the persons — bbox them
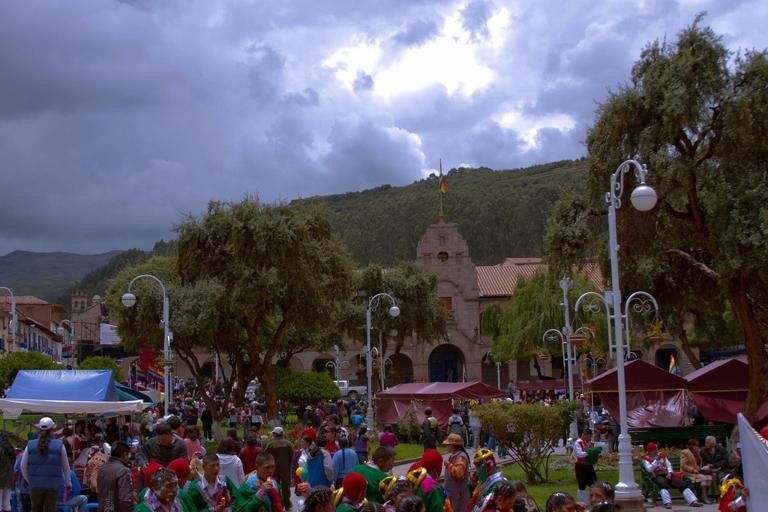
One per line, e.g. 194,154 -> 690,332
1,345 -> 767,512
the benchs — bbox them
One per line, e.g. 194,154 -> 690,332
639,457 -> 702,504
626,424 -> 732,449
229,414 -> 263,429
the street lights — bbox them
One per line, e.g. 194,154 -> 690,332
371,327 -> 396,393
580,349 -> 606,377
358,293 -> 399,433
56,320 -> 75,371
484,352 -> 501,390
122,274 -> 172,415
324,344 -> 350,386
0,285 -> 17,351
573,156 -> 660,511
541,274 -> 596,410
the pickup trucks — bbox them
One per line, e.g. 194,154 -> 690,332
332,380 -> 367,400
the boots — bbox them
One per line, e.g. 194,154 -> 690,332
701,486 -> 712,504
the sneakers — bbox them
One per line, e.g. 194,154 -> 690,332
665,503 -> 671,509
690,501 -> 704,507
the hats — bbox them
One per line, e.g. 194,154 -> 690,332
271,427 -> 283,435
40,417 -> 55,431
331,433 -> 494,503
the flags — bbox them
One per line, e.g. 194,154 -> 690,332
439,159 -> 448,193
